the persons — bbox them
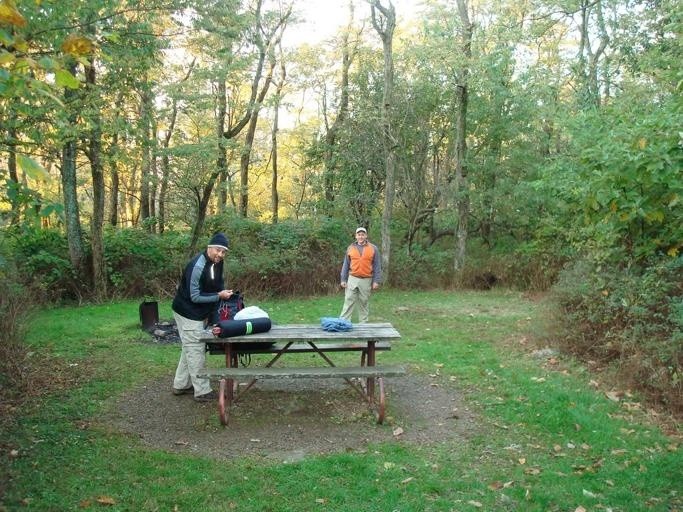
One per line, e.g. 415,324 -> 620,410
340,227 -> 381,322
171,232 -> 234,401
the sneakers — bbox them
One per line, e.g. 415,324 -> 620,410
194,390 -> 219,402
172,385 -> 194,396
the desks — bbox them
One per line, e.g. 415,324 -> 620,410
199,321 -> 403,401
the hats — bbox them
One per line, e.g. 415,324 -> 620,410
208,233 -> 229,251
356,227 -> 368,234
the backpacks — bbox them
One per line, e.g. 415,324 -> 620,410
209,288 -> 246,326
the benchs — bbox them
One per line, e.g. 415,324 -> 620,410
196,366 -> 407,426
208,340 -> 392,389
